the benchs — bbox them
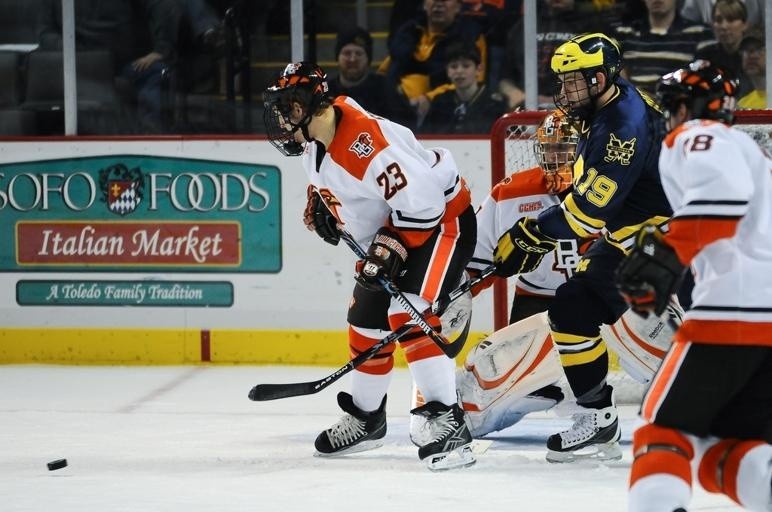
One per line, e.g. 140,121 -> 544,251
0,0 -> 772,134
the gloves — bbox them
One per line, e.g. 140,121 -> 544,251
493,216 -> 559,280
353,225 -> 414,295
614,226 -> 693,319
302,186 -> 343,246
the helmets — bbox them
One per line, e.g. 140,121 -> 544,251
649,58 -> 742,140
532,107 -> 584,194
260,57 -> 334,158
550,31 -> 625,111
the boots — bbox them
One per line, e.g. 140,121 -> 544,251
547,386 -> 623,453
314,390 -> 390,454
411,389 -> 474,462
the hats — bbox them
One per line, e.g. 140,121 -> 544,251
333,23 -> 372,62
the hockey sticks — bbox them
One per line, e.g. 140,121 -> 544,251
248,260 -> 501,402
335,227 -> 472,360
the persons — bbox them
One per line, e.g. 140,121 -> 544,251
451,107 -> 656,443
494,29 -> 695,452
611,59 -> 772,512
266,60 -> 472,458
